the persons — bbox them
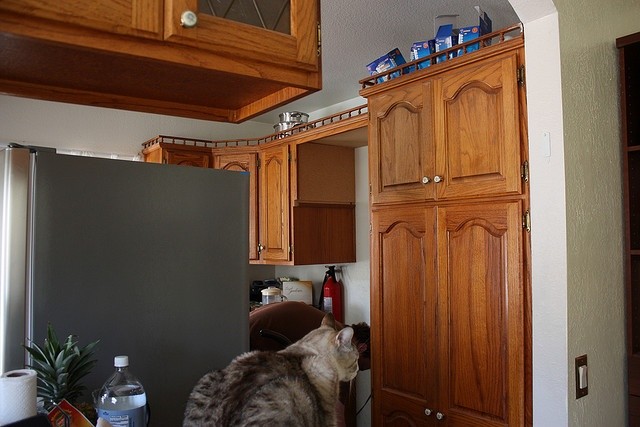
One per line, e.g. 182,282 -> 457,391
249,301 -> 371,427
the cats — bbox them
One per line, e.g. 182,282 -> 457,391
181,310 -> 360,427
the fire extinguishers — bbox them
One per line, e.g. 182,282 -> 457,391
318,265 -> 342,323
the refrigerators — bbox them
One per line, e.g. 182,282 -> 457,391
0,149 -> 251,427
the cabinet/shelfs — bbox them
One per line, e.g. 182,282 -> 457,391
0,0 -> 323,124
358,21 -> 531,205
210,147 -> 259,265
371,198 -> 532,427
146,144 -> 211,170
259,146 -> 357,267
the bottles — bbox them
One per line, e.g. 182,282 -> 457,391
98,355 -> 146,427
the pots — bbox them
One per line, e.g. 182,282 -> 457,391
278,110 -> 309,123
272,122 -> 316,139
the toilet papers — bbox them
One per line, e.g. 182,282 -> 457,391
0,368 -> 36,426
